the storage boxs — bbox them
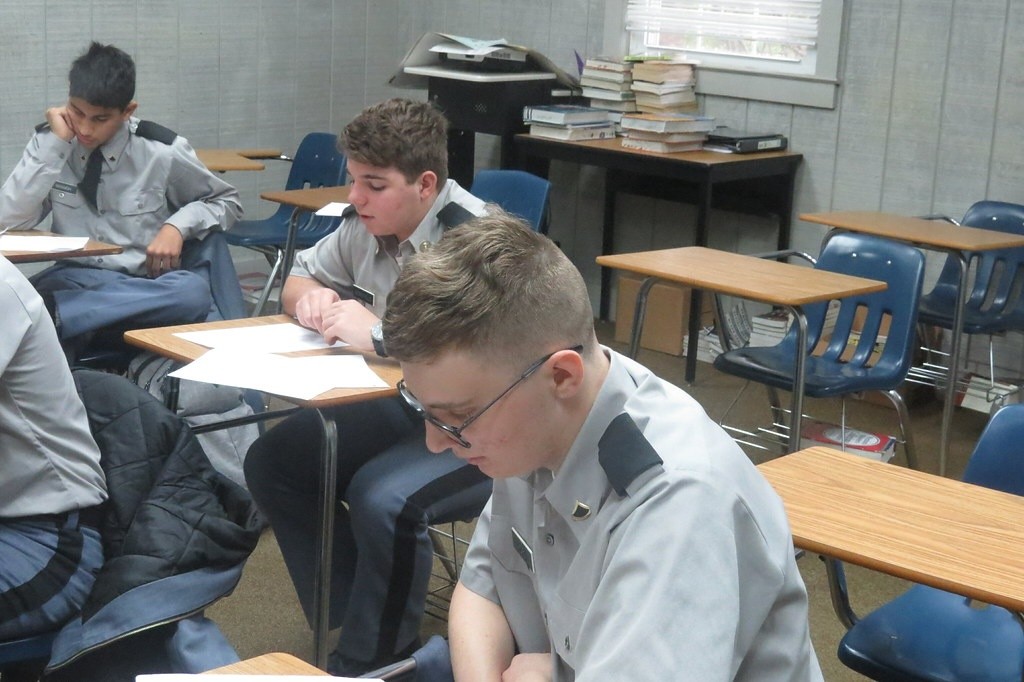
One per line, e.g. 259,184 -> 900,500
615,271 -> 714,356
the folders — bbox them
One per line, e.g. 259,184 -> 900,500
703,126 -> 788,154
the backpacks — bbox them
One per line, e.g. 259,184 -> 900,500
120,350 -> 270,533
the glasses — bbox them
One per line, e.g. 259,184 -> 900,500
396,344 -> 583,449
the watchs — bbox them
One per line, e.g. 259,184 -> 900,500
371,319 -> 390,358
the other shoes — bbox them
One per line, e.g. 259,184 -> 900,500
326,634 -> 421,681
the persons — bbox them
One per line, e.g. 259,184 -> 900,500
382,209 -> 826,682
0,38 -> 242,350
242,96 -> 507,676
0,249 -> 109,646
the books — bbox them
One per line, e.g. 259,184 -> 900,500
680,315 -> 886,365
523,52 -> 790,154
799,415 -> 898,463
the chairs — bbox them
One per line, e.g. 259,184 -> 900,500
76,231 -> 245,364
471,169 -> 551,234
224,131 -> 347,316
709,232 -> 919,472
916,200 -> 1024,401
837,404 -> 1024,682
0,370 -> 260,682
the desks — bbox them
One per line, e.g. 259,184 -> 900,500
507,132 -> 803,384
0,138 -> 1023,682
404,65 -> 584,191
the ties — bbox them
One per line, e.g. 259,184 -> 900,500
77,148 -> 106,211
